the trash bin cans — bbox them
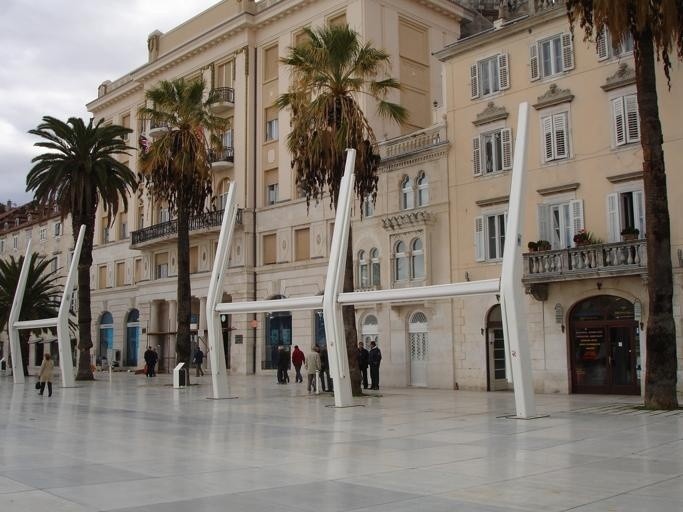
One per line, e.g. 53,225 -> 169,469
101,356 -> 107,372
173,362 -> 187,389
0,357 -> 7,376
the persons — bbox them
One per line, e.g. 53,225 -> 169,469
315,343 -> 332,391
356,341 -> 369,389
36,352 -> 54,397
148,348 -> 158,377
192,346 -> 203,377
303,345 -> 320,393
291,345 -> 305,383
366,340 -> 381,390
142,345 -> 156,377
274,345 -> 290,384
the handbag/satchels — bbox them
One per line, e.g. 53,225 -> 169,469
35,382 -> 41,390
